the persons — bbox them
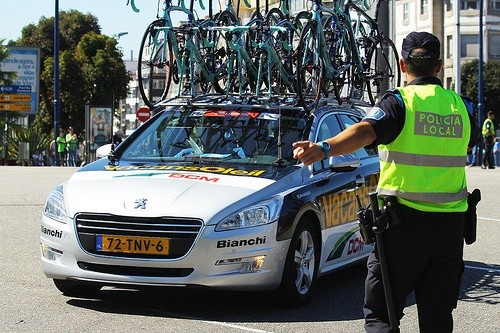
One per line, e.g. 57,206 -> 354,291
481,111 -> 497,169
492,138 -> 500,167
56,131 -> 66,167
50,139 -> 56,166
292,31 -> 481,333
65,127 -> 79,167
465,142 -> 482,167
107,129 -> 125,144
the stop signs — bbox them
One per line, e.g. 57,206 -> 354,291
136,107 -> 151,122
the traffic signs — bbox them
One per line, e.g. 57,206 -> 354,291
0,84 -> 32,112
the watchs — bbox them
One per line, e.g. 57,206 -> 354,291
318,141 -> 330,159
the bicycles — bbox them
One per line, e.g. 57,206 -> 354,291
126,0 -> 400,113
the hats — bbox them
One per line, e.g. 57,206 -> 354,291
401,31 -> 440,60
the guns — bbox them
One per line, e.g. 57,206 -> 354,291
465,189 -> 481,212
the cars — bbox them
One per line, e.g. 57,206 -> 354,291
37,88 -> 385,304
